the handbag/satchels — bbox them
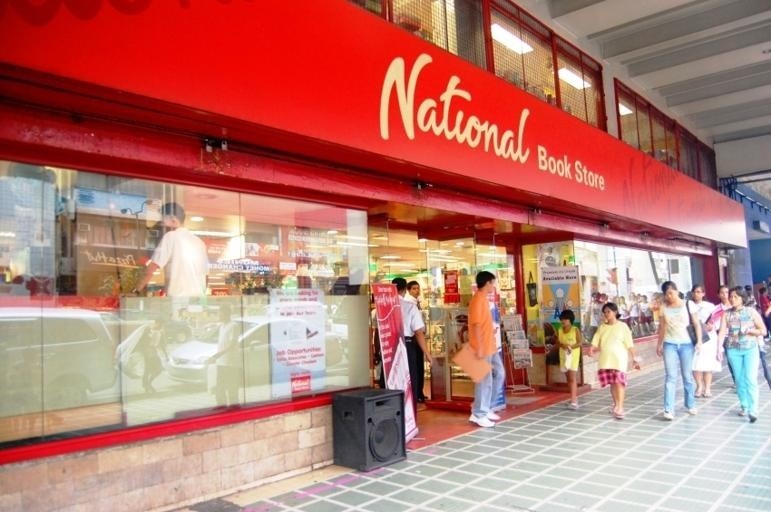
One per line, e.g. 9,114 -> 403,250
526,271 -> 538,307
686,299 -> 709,346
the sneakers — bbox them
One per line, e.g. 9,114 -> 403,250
487,411 -> 500,420
663,412 -> 673,419
468,414 -> 494,427
687,408 -> 697,415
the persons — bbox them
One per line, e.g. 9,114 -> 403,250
655,280 -> 703,422
115,199 -> 211,297
716,285 -> 768,423
584,290 -> 656,344
138,317 -> 170,397
206,302 -> 242,414
406,280 -> 430,405
467,271 -> 506,431
688,277 -> 771,398
556,309 -> 583,410
368,277 -> 433,389
586,301 -> 642,419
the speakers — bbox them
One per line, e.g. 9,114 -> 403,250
332,388 -> 407,473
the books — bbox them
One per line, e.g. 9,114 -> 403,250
452,344 -> 496,387
502,314 -> 533,370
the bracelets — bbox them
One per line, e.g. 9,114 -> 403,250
130,287 -> 142,296
